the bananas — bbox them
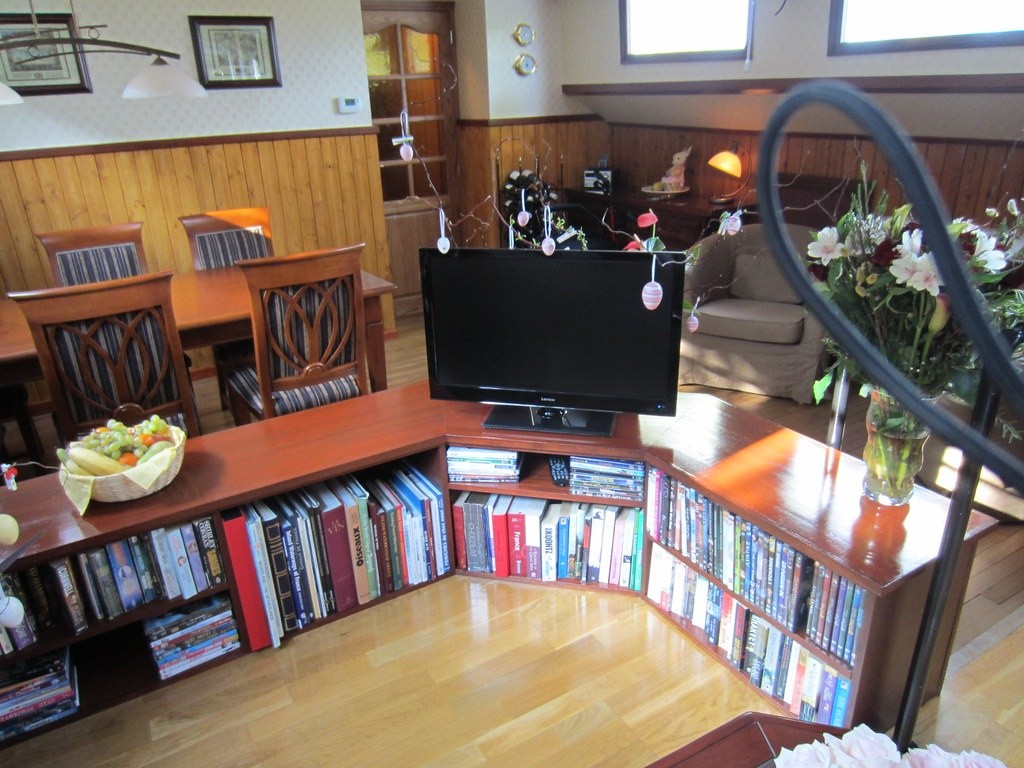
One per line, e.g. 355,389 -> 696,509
64,446 -> 134,476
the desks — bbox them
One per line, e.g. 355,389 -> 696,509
565,186 -> 756,246
0,267 -> 396,391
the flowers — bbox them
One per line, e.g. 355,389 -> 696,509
797,162 -> 1024,497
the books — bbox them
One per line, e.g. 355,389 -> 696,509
0,517 -> 226,659
646,542 -> 854,727
447,446 -> 524,483
569,456 -> 645,501
646,466 -> 866,668
453,492 -> 644,590
0,646 -> 79,742
145,592 -> 240,680
222,464 -> 451,651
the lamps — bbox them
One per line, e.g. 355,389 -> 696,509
0,24 -> 209,99
0,82 -> 25,105
708,140 -> 751,204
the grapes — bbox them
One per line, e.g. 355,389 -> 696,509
79,414 -> 171,460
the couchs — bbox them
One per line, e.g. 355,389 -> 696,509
680,222 -> 832,404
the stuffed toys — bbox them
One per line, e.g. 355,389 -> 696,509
663,146 -> 692,191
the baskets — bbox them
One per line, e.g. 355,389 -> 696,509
89,424 -> 186,503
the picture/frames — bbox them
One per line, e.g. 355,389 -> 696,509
189,15 -> 282,89
0,13 -> 93,96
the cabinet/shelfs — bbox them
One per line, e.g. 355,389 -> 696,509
641,460 -> 874,727
0,440 -> 449,749
447,443 -> 648,595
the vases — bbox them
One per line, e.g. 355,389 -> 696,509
863,383 -> 931,508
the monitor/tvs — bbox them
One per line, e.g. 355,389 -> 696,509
418,247 -> 686,437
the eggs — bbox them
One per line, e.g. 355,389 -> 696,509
0,514 -> 19,545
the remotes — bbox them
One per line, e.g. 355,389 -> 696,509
549,455 -> 570,487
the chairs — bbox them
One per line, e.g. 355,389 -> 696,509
0,381 -> 46,470
178,206 -> 274,410
223,240 -> 369,427
7,268 -> 199,440
34,223 -> 151,287
